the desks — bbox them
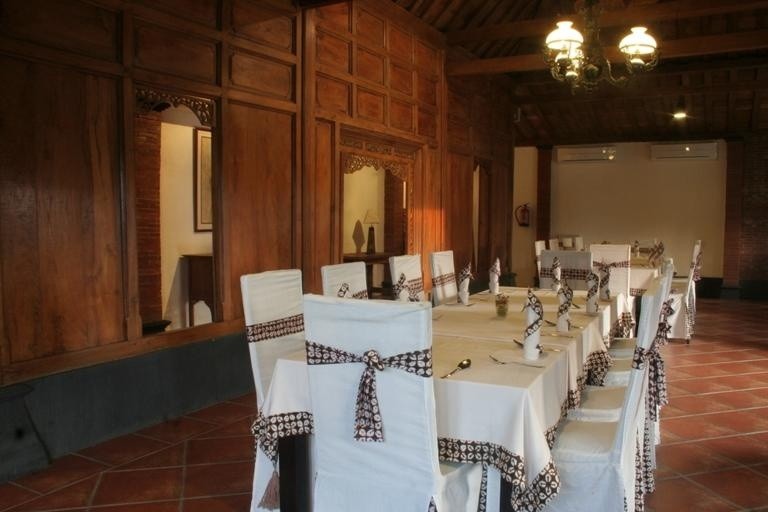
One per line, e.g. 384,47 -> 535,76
183,253 -> 215,327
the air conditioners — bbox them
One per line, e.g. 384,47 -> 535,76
648,142 -> 718,161
555,145 -> 616,164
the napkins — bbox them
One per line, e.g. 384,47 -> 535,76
338,283 -> 353,300
488,256 -> 502,296
395,272 -> 411,301
556,275 -> 574,334
522,287 -> 544,362
457,262 -> 475,306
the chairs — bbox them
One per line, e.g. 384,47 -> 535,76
305,292 -> 501,512
540,348 -> 656,512
320,261 -> 369,299
534,236 -> 701,345
430,250 -> 457,307
568,256 -> 676,493
238,268 -> 307,415
389,254 -> 426,301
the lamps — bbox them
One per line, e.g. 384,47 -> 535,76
539,0 -> 663,97
363,208 -> 381,253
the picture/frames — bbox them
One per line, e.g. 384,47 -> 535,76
192,125 -> 215,231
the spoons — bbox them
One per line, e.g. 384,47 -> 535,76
440,358 -> 471,379
